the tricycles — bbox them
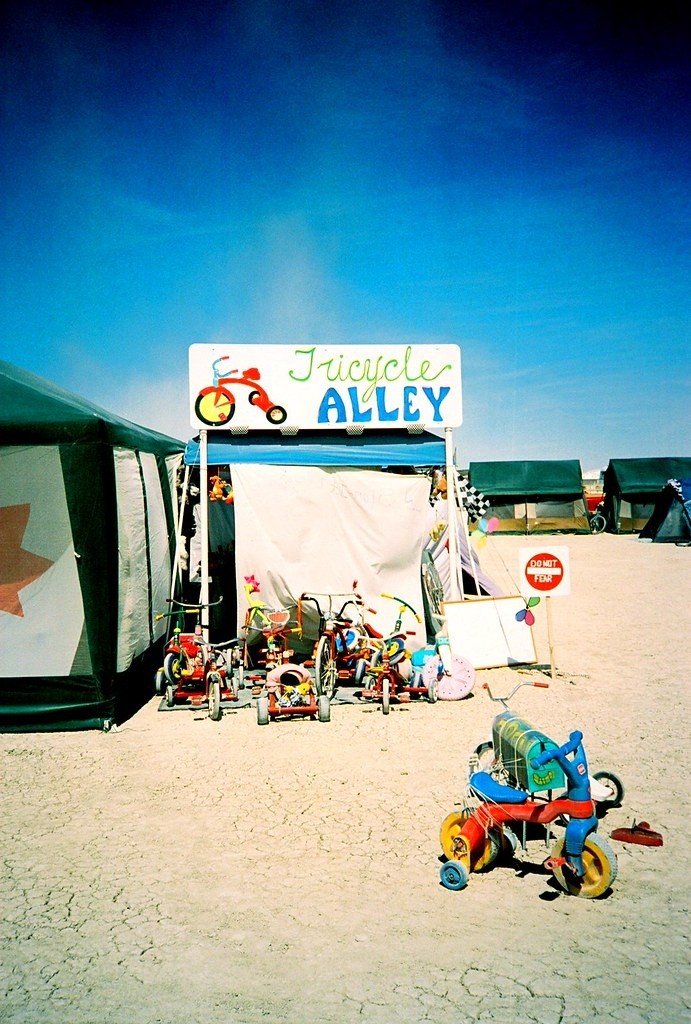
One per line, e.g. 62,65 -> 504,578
438,679 -> 625,898
153,581 -> 438,725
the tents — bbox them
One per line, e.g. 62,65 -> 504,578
602,455 -> 691,535
468,458 -> 592,536
182,427 -> 457,682
637,478 -> 691,543
0,360 -> 187,734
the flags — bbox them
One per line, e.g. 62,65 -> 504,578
455,471 -> 490,525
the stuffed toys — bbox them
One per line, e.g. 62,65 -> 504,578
207,474 -> 234,504
433,473 -> 447,499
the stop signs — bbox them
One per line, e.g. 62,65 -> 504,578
519,544 -> 572,598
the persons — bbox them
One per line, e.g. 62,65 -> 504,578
426,465 -> 444,481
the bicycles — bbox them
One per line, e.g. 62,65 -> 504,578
590,506 -> 607,534
